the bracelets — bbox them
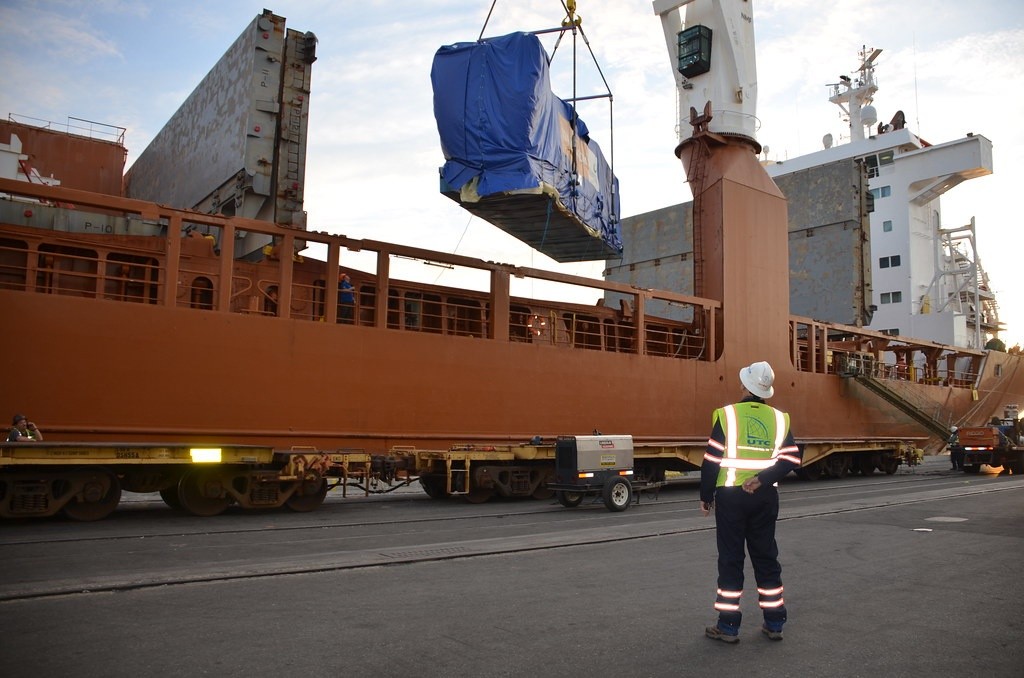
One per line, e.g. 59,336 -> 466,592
32,427 -> 38,432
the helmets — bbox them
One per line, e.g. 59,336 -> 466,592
739,361 -> 776,399
951,426 -> 958,432
339,274 -> 346,280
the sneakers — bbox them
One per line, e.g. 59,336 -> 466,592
706,611 -> 742,643
762,605 -> 788,640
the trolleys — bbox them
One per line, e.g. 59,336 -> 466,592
545,430 -> 669,513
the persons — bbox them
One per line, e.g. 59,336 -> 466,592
339,273 -> 358,323
9,415 -> 43,441
700,361 -> 800,643
950,426 -> 966,471
266,293 -> 276,314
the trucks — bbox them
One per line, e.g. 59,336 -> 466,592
958,405 -> 1023,477
